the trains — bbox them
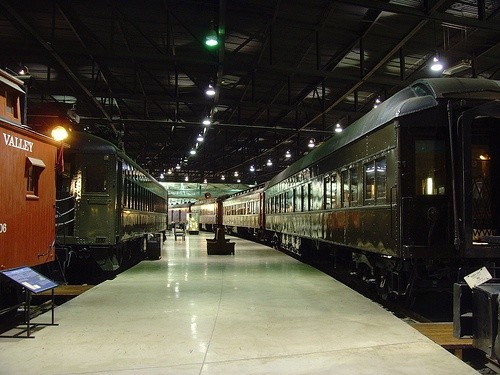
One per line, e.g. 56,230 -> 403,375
0,63 -> 170,317
168,76 -> 500,320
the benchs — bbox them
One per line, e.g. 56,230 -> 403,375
207,227 -> 236,255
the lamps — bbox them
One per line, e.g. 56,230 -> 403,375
202,108 -> 211,126
431,21 -> 443,71
206,78 -> 215,95
205,18 -> 218,47
